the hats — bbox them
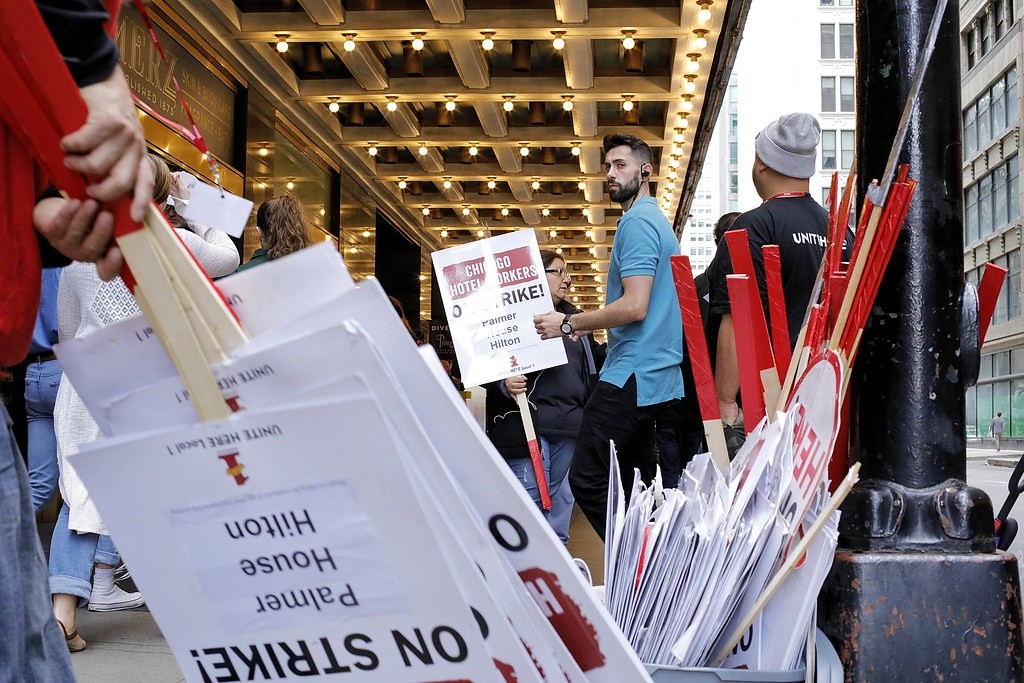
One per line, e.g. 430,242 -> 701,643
756,112 -> 820,178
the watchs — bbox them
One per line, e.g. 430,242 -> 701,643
561,314 -> 575,335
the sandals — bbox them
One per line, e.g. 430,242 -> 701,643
55,619 -> 87,653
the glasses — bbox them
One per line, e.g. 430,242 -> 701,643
543,267 -> 573,282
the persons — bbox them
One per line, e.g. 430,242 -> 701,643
235,195 -> 312,273
88,535 -> 144,612
0,0 -> 155,683
990,412 -> 1004,452
533,134 -> 685,585
657,111 -> 856,488
48,153 -> 240,652
479,249 -> 598,547
24,267 -> 63,515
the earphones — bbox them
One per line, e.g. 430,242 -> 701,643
642,172 -> 650,177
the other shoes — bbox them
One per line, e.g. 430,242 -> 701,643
90,563 -> 131,582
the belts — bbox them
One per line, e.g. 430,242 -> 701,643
25,350 -> 58,363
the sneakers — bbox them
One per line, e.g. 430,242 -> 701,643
86,585 -> 146,613
